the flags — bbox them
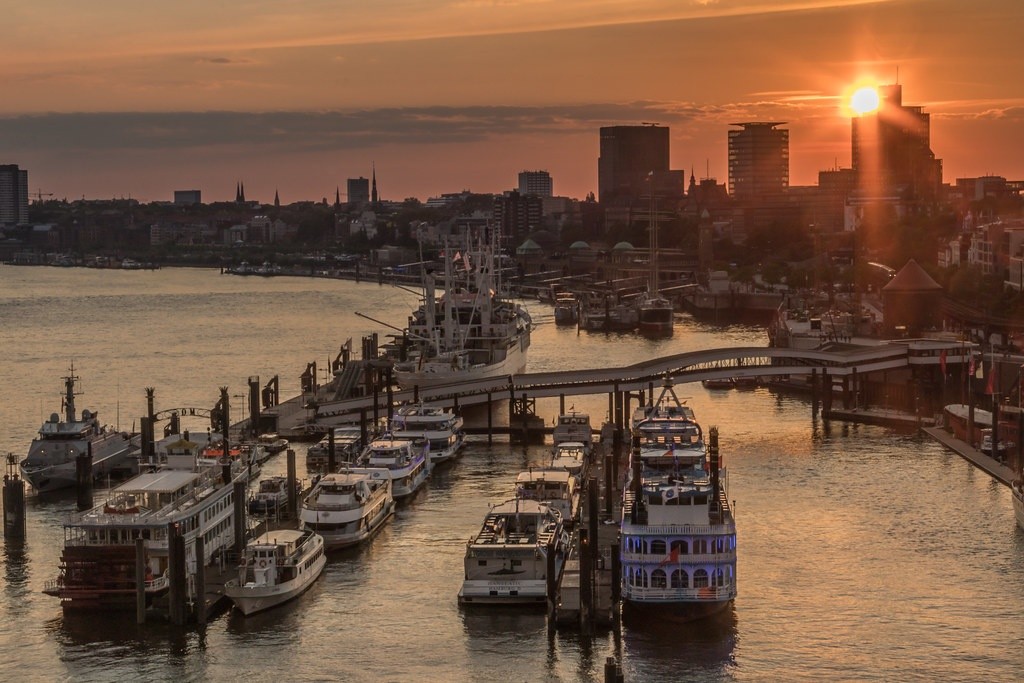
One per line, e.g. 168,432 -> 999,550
439,248 -> 450,257
451,251 -> 462,262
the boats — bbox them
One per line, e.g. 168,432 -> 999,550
944,404 -> 1007,447
454,410 -> 596,611
296,465 -> 396,550
16,369 -> 144,494
38,431 -> 291,616
1010,478 -> 1024,530
619,363 -> 738,607
303,426 -> 362,473
221,528 -> 327,620
355,218 -> 533,413
246,477 -> 304,517
390,402 -> 469,469
555,292 -> 675,332
355,431 -> 435,503
